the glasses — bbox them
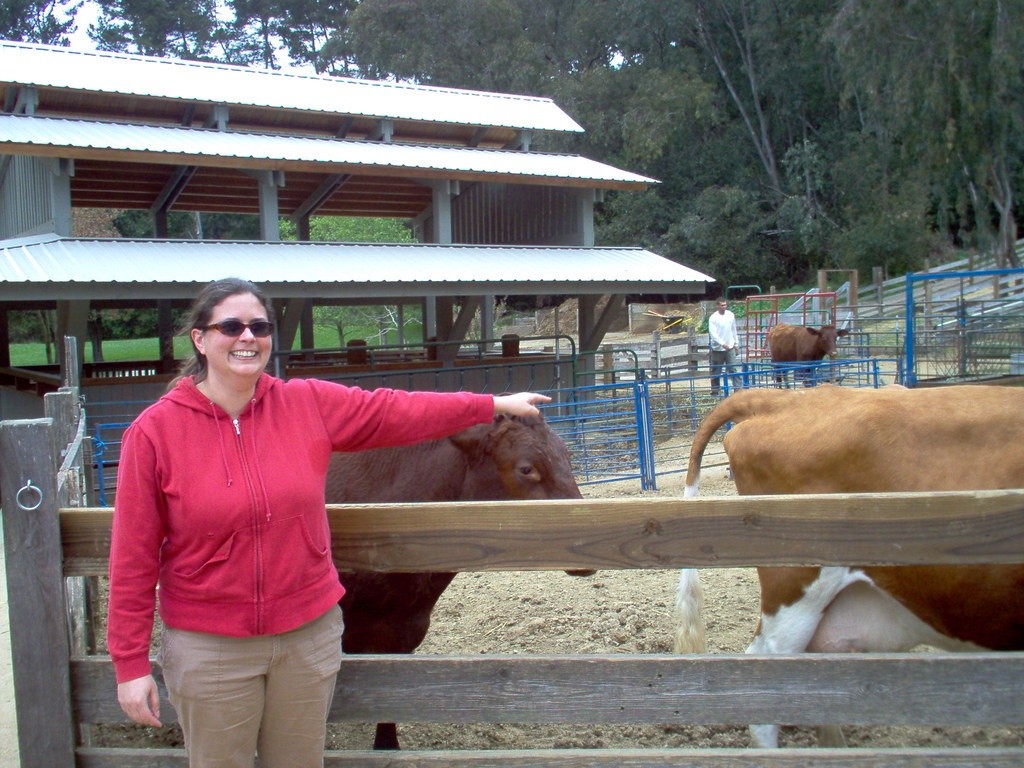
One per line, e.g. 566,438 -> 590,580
202,319 -> 277,338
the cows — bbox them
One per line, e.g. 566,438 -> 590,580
326,412 -> 597,751
667,382 -> 1024,748
760,315 -> 856,390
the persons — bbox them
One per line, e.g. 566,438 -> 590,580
709,296 -> 742,395
107,278 -> 552,768
